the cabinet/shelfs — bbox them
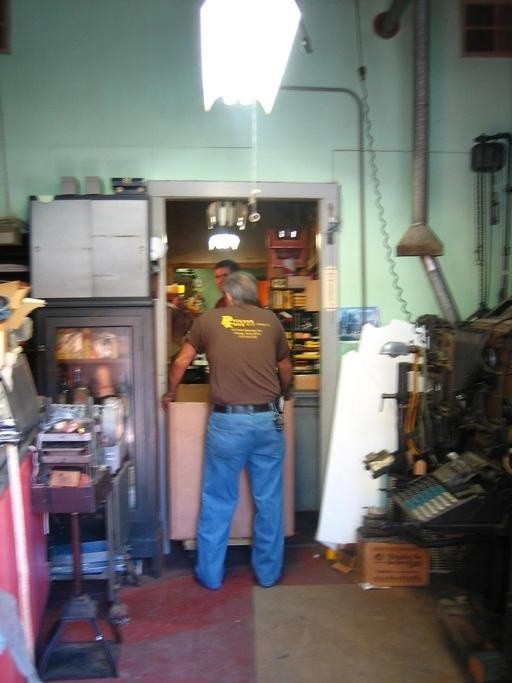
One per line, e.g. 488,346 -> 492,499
33,296 -> 158,575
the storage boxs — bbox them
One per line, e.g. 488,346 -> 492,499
357,535 -> 434,589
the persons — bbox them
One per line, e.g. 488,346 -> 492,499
214,259 -> 243,309
159,268 -> 294,592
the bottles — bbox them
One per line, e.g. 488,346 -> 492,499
114,368 -> 133,418
56,364 -> 82,404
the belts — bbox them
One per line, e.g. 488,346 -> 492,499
212,403 -> 272,413
